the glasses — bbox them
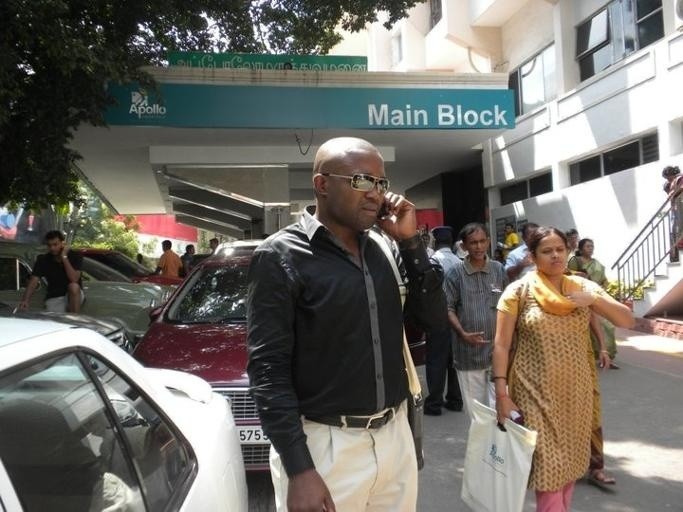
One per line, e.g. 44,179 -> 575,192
323,173 -> 390,197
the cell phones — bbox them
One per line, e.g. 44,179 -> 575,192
377,202 -> 390,216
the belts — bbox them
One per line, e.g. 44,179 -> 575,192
304,395 -> 406,429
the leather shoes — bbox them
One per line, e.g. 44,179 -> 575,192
424,400 -> 463,415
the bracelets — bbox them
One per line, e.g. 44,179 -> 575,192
490,375 -> 508,382
60,255 -> 68,259
494,393 -> 507,398
598,349 -> 610,355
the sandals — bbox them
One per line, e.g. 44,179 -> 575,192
589,469 -> 615,485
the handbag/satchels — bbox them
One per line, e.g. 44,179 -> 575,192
460,398 -> 538,512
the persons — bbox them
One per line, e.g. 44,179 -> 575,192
663,181 -> 678,262
19,230 -> 86,315
403,263 -> 462,415
417,227 -> 470,307
568,239 -> 619,369
242,136 -> 449,511
564,230 -> 579,249
496,224 -> 539,281
662,166 -> 682,250
443,222 -> 506,418
562,237 -> 616,486
135,236 -> 219,281
488,225 -> 636,511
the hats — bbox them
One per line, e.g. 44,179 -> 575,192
430,226 -> 454,241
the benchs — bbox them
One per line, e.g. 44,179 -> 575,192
0,395 -> 110,512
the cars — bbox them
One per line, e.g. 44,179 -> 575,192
1,237 -> 271,383
1,316 -> 252,511
131,251 -> 271,473
